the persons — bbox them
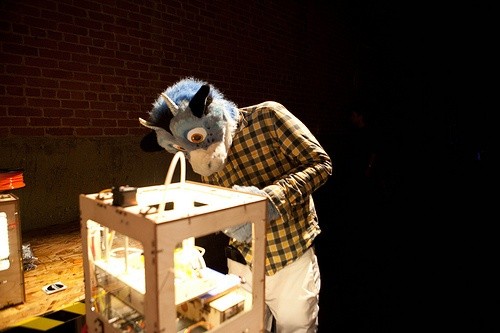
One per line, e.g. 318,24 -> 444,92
344,109 -> 374,223
138,77 -> 333,333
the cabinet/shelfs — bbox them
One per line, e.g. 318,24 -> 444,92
0,193 -> 26,313
78,179 -> 267,333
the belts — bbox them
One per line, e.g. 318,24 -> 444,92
224,245 -> 247,266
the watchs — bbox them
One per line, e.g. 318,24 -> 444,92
366,163 -> 373,169
